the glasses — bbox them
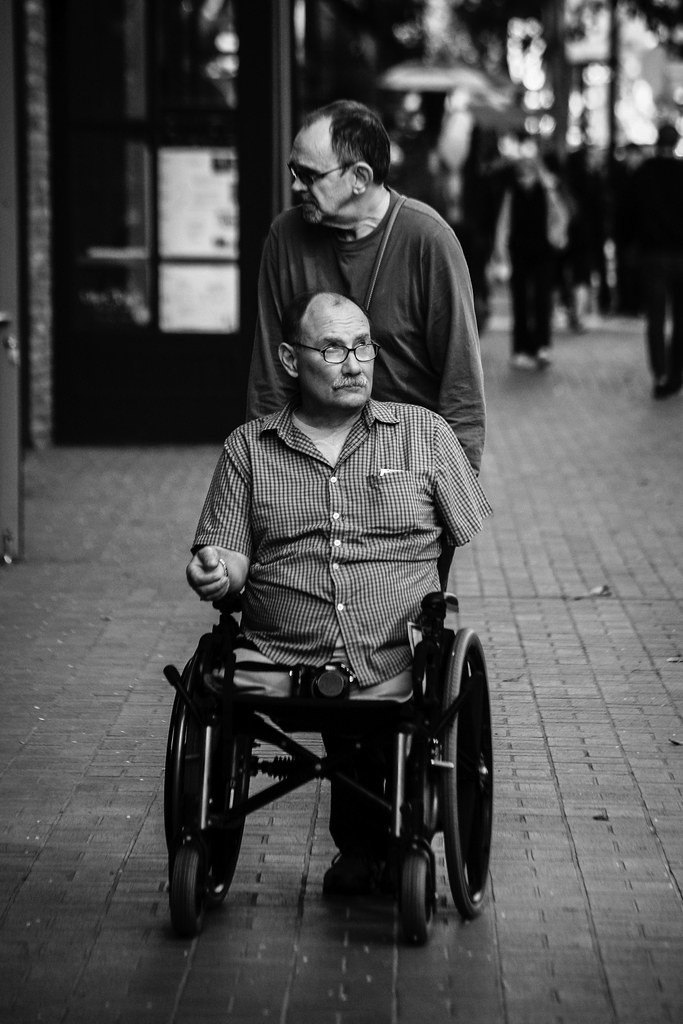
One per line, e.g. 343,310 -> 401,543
290,342 -> 380,364
285,161 -> 344,185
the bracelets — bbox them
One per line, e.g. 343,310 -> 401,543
219,558 -> 228,577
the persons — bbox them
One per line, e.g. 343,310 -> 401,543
247,99 -> 487,905
399,121 -> 682,398
184,292 -> 493,703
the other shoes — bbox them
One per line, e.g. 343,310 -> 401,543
323,853 -> 384,898
